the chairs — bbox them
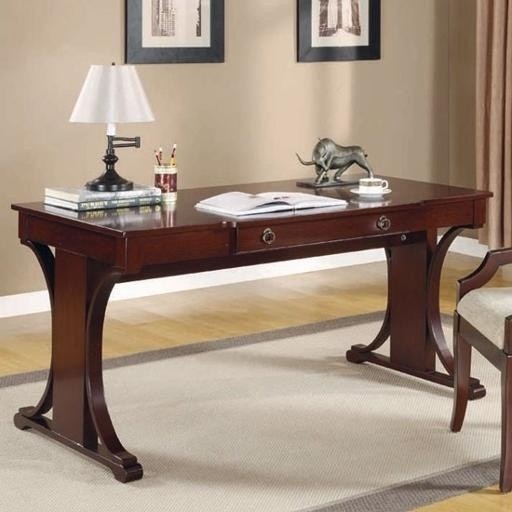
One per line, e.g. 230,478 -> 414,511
448,247 -> 511,497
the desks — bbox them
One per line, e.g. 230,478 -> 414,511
11,175 -> 494,487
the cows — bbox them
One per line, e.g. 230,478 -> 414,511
295,137 -> 373,185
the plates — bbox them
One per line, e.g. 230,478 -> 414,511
349,188 -> 392,199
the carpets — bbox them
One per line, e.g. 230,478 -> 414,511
0,307 -> 512,511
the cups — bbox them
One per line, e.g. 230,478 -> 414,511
153,163 -> 178,202
359,178 -> 387,194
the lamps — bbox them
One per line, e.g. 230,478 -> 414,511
69,62 -> 156,192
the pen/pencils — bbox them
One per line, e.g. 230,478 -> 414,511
171,144 -> 177,166
154,150 -> 161,166
159,147 -> 163,166
274,196 -> 289,199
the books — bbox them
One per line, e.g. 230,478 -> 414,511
193,191 -> 349,217
196,206 -> 348,221
44,205 -> 162,218
44,196 -> 162,212
82,216 -> 162,225
44,186 -> 162,204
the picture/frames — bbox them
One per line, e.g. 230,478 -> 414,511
297,1 -> 382,61
126,0 -> 224,64
